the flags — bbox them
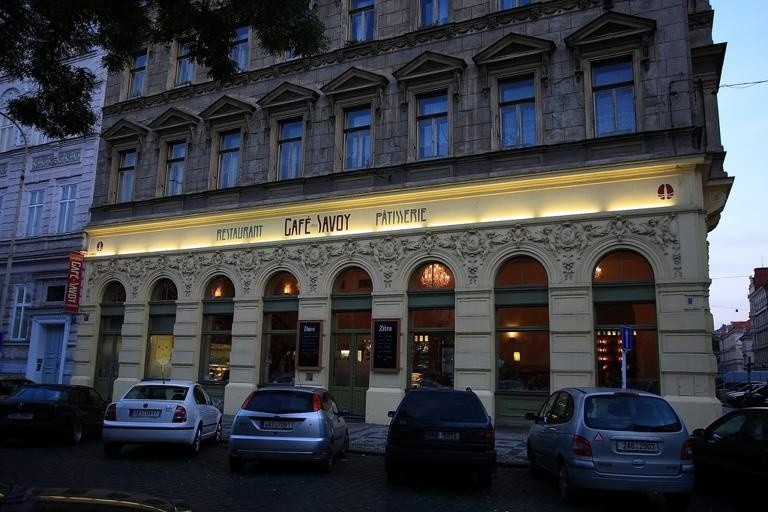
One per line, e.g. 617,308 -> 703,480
62,248 -> 84,313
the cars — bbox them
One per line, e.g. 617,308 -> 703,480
524,387 -> 695,512
228,375 -> 349,473
688,380 -> 768,512
0,377 -> 112,446
384,386 -> 497,489
102,367 -> 224,461
